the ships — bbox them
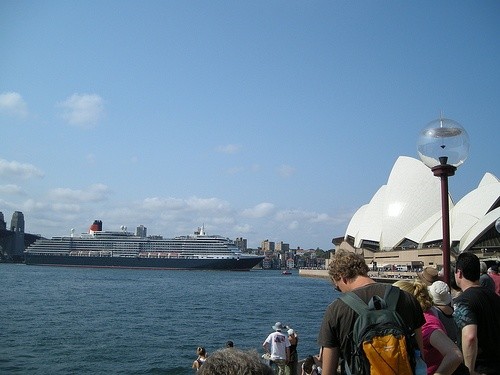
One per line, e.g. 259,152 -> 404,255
21,224 -> 267,272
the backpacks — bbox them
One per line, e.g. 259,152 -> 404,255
337,285 -> 415,375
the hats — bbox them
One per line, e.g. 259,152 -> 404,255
272,322 -> 285,331
418,266 -> 438,285
427,281 -> 451,305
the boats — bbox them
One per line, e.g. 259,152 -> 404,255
282,269 -> 292,275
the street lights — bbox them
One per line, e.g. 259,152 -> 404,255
416,110 -> 472,307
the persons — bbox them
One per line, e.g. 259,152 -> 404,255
191,346 -> 208,372
391,280 -> 464,375
287,329 -> 298,375
226,341 -> 233,348
421,261 -> 500,350
452,252 -> 500,375
302,355 -> 322,375
367,267 -> 424,280
300,266 -> 329,270
318,252 -> 426,375
263,322 -> 291,375
